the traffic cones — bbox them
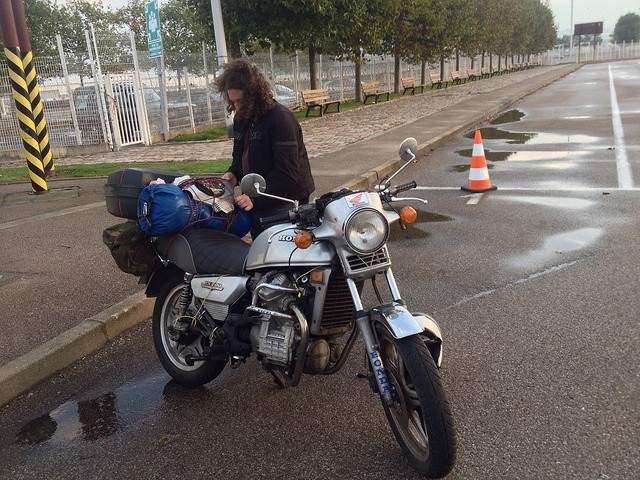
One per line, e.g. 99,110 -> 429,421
461,128 -> 498,193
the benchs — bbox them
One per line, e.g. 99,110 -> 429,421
481,62 -> 541,79
362,82 -> 390,104
450,71 -> 467,84
401,77 -> 425,95
301,88 -> 340,117
467,69 -> 481,81
430,74 -> 448,89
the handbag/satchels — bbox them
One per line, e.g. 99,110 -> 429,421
102,219 -> 158,276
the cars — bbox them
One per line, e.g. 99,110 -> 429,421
273,85 -> 302,106
323,78 -> 367,98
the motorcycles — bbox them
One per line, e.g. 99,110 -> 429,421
103,135 -> 457,480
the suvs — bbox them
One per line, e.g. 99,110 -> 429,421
68,81 -> 196,140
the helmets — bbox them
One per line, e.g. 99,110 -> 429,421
397,312 -> 444,405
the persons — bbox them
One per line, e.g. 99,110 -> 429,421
211,57 -> 316,242
